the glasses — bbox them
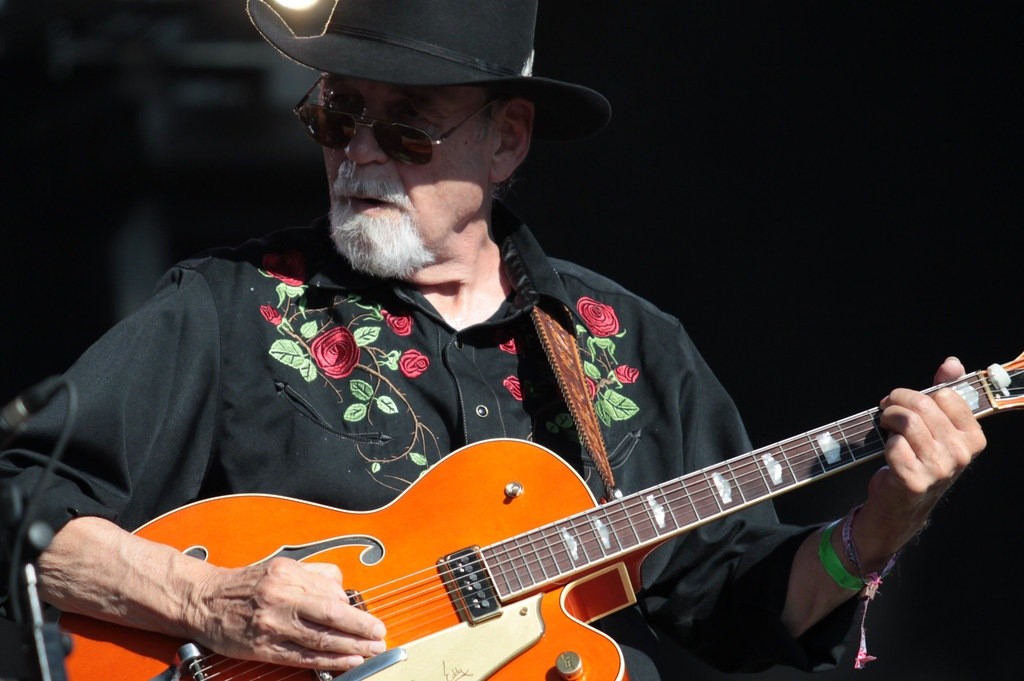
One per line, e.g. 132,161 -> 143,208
292,72 -> 505,165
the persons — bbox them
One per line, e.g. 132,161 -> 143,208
0,0 -> 987,681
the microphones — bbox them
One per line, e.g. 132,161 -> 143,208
0,376 -> 67,443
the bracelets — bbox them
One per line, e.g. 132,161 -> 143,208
819,517 -> 869,591
843,504 -> 902,669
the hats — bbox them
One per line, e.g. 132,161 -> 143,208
247,0 -> 611,144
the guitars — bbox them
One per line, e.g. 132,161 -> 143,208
60,357 -> 1022,681
